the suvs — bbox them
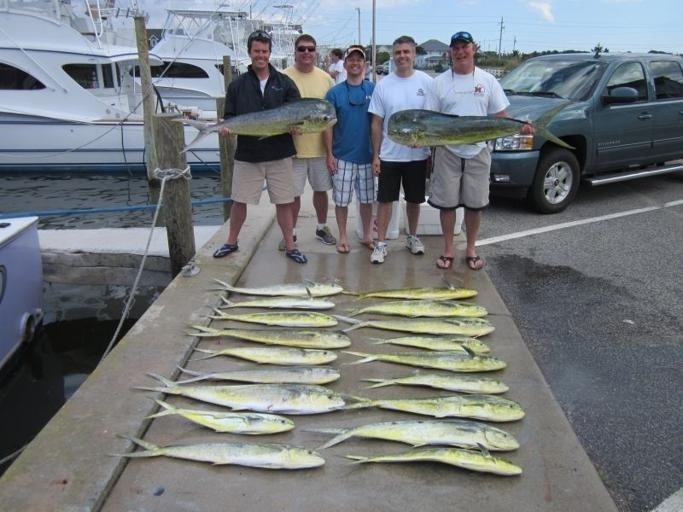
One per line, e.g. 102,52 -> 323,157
489,51 -> 682,209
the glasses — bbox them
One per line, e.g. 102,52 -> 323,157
248,33 -> 271,48
348,98 -> 366,106
296,46 -> 315,52
453,34 -> 470,39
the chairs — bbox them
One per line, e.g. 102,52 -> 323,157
611,87 -> 639,96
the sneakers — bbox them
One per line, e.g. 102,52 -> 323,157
277,226 -> 485,270
213,241 -> 238,257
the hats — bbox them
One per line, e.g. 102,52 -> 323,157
450,32 -> 473,47
344,45 -> 365,58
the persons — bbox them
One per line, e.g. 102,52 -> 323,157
328,48 -> 348,84
408,31 -> 536,271
212,30 -> 308,264
321,44 -> 377,254
278,34 -> 337,250
367,36 -> 434,264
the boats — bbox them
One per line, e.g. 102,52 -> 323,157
0,0 -> 308,172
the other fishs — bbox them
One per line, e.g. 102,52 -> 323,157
108,277 -> 526,476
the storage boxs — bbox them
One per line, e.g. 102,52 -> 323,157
356,177 -> 404,240
402,195 -> 463,236
